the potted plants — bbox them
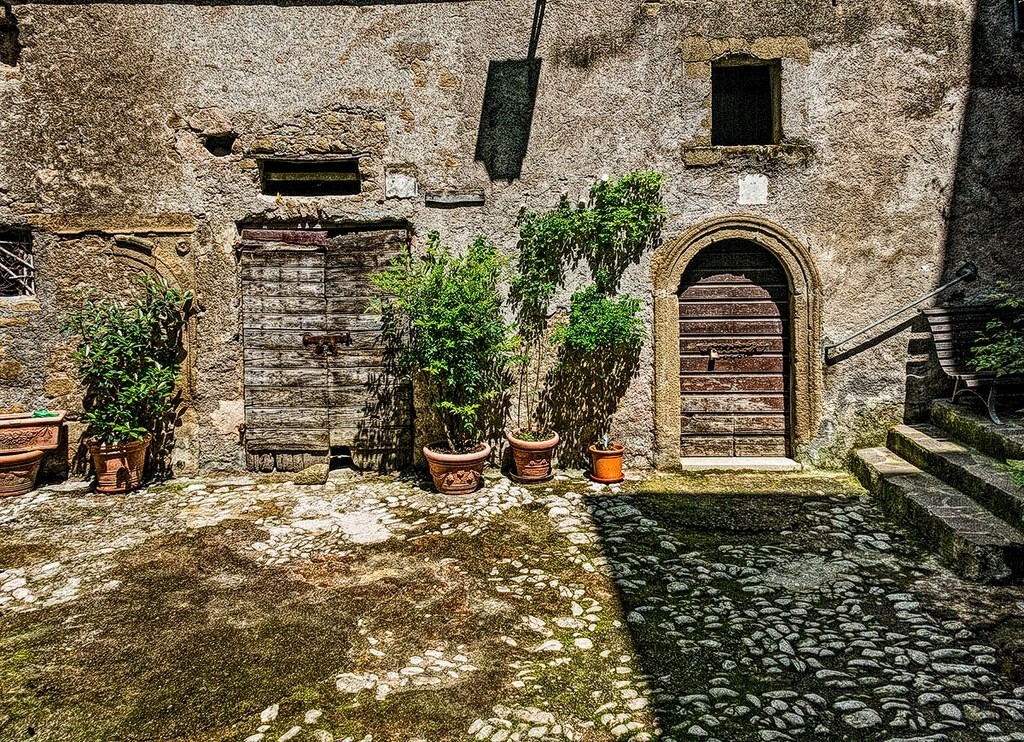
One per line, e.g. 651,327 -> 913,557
58,270 -> 202,490
372,227 -> 546,495
546,282 -> 648,486
500,191 -> 626,482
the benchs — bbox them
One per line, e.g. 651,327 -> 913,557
917,302 -> 1024,425
